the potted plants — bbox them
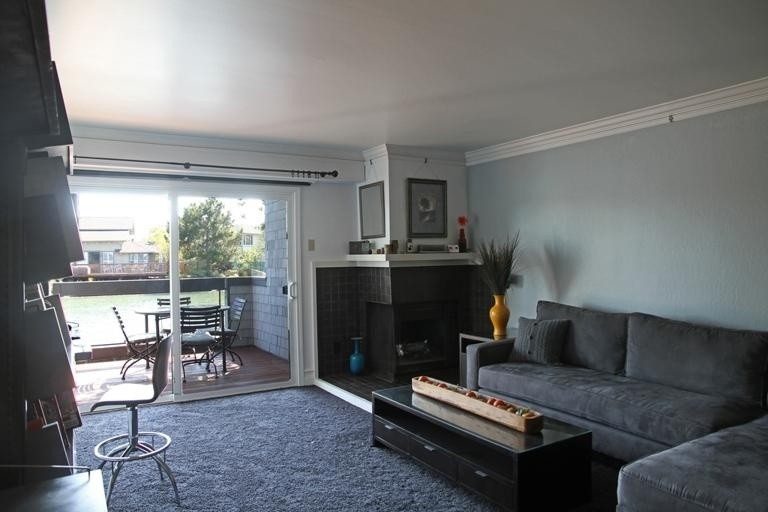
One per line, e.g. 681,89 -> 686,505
408,178 -> 448,238
477,227 -> 523,336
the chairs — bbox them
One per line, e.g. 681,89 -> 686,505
111,307 -> 164,381
181,305 -> 221,383
89,331 -> 182,509
157,296 -> 196,362
199,297 -> 247,370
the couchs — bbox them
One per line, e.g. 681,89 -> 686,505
465,299 -> 768,466
615,414 -> 768,512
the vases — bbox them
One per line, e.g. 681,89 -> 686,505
459,227 -> 466,252
349,336 -> 367,374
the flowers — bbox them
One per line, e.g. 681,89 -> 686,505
457,214 -> 468,228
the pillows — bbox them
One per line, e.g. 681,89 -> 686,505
508,316 -> 570,368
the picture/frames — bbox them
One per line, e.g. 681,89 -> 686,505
358,180 -> 386,240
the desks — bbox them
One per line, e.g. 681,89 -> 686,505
135,305 -> 231,372
